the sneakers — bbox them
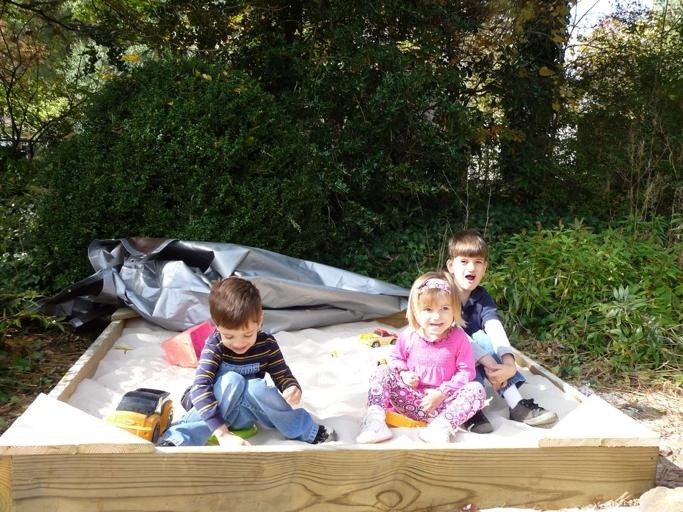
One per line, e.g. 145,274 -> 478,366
509,398 -> 557,426
312,425 -> 335,444
418,428 -> 450,444
156,441 -> 175,446
357,422 -> 392,443
463,410 -> 491,433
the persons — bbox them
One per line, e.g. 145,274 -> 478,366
356,271 -> 486,443
445,230 -> 557,434
156,277 -> 337,446
